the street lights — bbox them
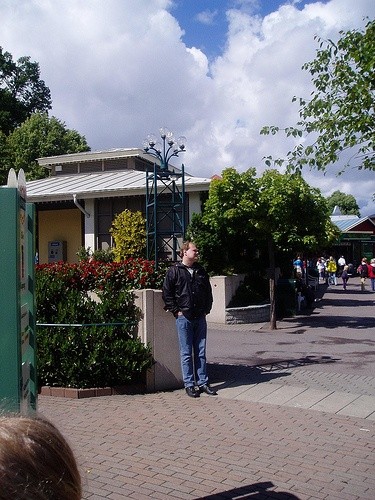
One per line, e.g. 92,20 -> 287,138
144,128 -> 186,275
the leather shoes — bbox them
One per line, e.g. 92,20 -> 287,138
199,384 -> 217,395
185,387 -> 200,398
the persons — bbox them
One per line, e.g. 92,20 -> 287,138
292,256 -> 375,292
162,240 -> 216,398
0,410 -> 89,500
